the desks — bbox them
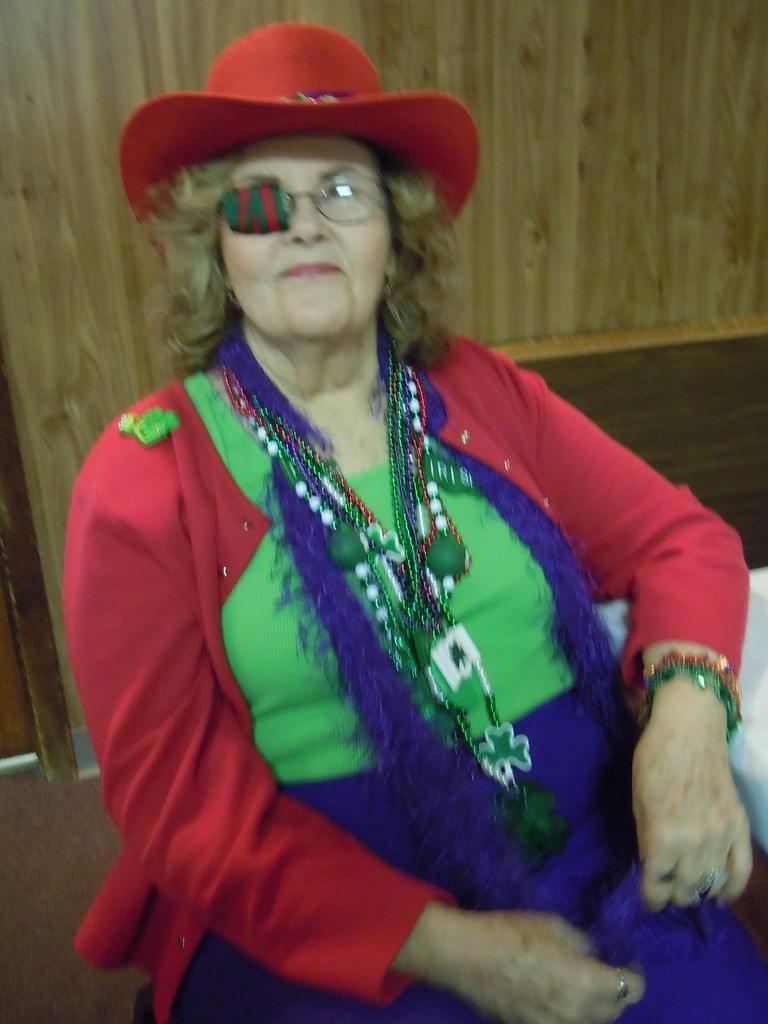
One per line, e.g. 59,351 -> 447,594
590,567 -> 768,847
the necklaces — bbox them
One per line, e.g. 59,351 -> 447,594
218,337 -> 564,861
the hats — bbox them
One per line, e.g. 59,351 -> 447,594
119,20 -> 478,256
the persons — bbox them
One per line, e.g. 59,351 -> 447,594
61,22 -> 768,1024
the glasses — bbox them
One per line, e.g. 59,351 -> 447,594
215,177 -> 388,235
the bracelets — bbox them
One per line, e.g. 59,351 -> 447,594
641,650 -> 742,735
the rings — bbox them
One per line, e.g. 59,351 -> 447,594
698,881 -> 712,898
615,968 -> 629,1001
